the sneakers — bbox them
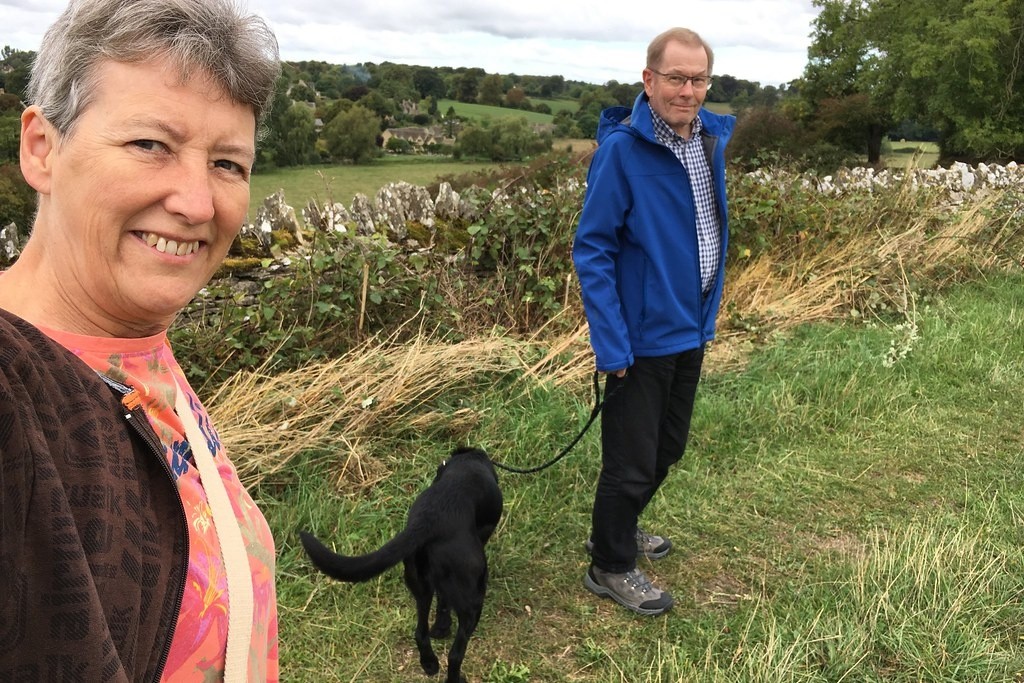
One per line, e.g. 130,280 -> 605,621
584,563 -> 673,617
585,526 -> 671,558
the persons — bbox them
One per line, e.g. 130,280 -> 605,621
0,0 -> 286,683
572,26 -> 737,616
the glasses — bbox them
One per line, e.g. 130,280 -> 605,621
646,65 -> 715,90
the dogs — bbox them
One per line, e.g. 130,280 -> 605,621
300,445 -> 504,683
